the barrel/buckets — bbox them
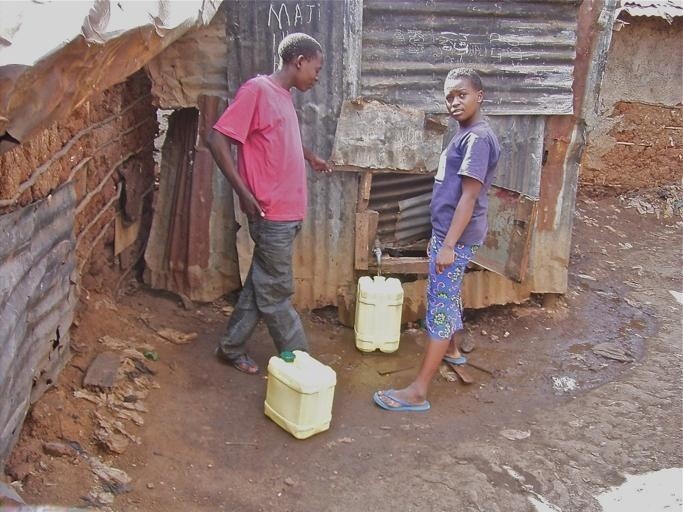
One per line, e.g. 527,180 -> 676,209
262,349 -> 338,439
353,274 -> 404,353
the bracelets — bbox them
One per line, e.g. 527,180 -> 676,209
443,241 -> 453,249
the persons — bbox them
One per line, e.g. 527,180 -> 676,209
372,67 -> 502,411
208,32 -> 332,374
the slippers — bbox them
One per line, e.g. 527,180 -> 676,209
216,347 -> 261,374
442,355 -> 467,366
373,388 -> 431,412
